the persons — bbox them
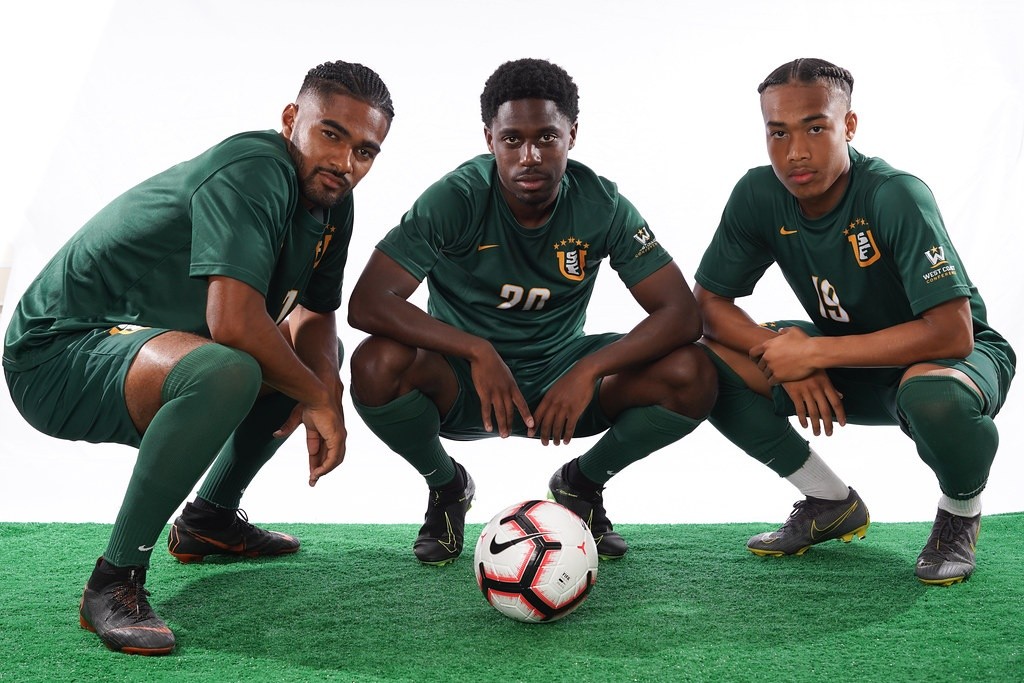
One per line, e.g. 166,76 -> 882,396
690,58 -> 1017,586
1,59 -> 394,653
347,59 -> 720,566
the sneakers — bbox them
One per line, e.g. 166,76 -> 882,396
547,464 -> 629,559
414,465 -> 476,565
916,507 -> 983,585
748,483 -> 871,556
79,556 -> 175,654
167,502 -> 300,563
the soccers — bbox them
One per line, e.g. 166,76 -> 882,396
474,501 -> 600,624
